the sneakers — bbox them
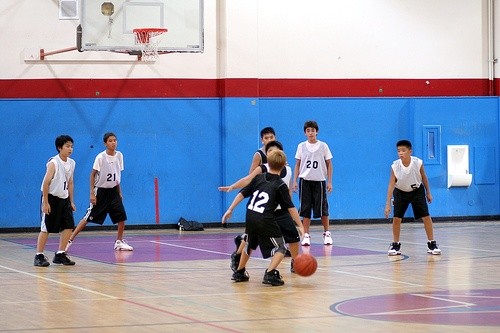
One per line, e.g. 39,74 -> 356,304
322,230 -> 333,245
65,240 -> 72,252
388,242 -> 401,255
262,269 -> 284,286
114,239 -> 133,251
34,254 -> 50,267
230,251 -> 241,272
231,267 -> 249,281
53,251 -> 75,265
427,239 -> 441,255
301,232 -> 311,245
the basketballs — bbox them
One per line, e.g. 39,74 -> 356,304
293,253 -> 317,278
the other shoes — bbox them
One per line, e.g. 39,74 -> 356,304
235,235 -> 242,251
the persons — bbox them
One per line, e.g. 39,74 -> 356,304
63,133 -> 135,252
386,139 -> 441,256
234,126 -> 292,257
291,120 -> 333,247
33,135 -> 76,267
218,141 -> 301,272
222,150 -> 305,285
